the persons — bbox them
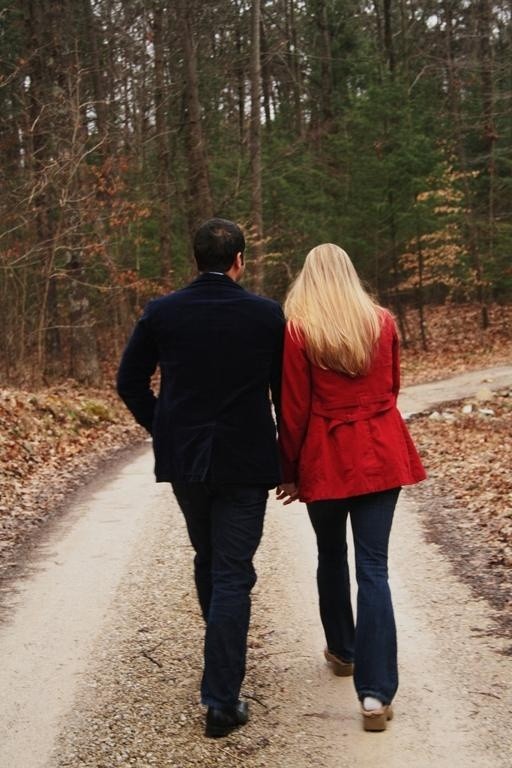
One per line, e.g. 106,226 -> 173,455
117,217 -> 286,738
271,243 -> 427,734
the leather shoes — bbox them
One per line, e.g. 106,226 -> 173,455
204,701 -> 249,738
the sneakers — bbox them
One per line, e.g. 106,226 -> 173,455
324,649 -> 354,677
361,704 -> 393,732
